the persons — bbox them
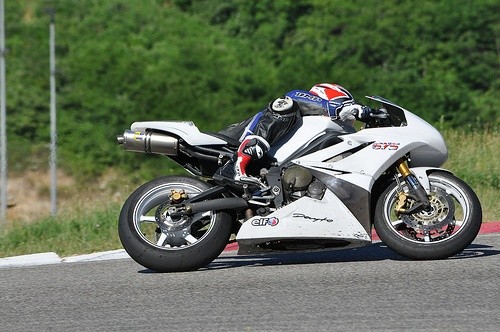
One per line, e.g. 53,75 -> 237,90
212,82 -> 371,192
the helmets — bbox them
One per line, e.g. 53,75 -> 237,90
308,82 -> 355,104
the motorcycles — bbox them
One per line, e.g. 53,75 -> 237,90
114,95 -> 484,273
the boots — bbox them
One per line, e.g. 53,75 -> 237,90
212,128 -> 270,196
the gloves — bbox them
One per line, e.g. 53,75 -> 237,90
326,101 -> 372,126
